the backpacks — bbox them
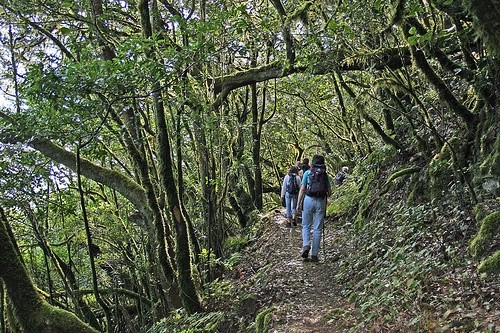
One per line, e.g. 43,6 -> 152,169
307,166 -> 326,192
288,175 -> 300,194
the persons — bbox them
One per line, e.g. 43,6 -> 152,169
281,166 -> 302,228
332,166 -> 349,187
296,158 -> 311,222
296,155 -> 331,262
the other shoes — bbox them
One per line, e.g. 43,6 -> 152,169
292,219 -> 297,226
312,256 -> 318,262
301,247 -> 309,258
287,221 -> 291,228
